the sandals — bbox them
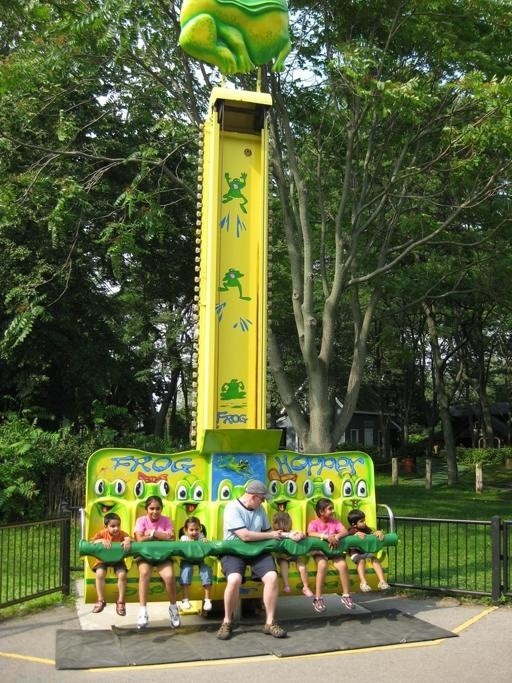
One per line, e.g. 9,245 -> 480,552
92,600 -> 106,613
116,599 -> 126,616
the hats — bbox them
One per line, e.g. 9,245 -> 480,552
245,480 -> 272,499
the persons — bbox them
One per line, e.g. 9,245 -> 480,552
347,509 -> 391,593
272,510 -> 313,598
132,495 -> 181,631
88,513 -> 136,616
215,480 -> 306,640
306,498 -> 356,613
178,516 -> 213,611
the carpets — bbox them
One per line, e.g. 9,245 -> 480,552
239,605 -> 461,659
50,620 -> 273,671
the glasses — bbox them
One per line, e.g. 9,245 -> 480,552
255,495 -> 264,502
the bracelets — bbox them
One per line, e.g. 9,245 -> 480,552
150,529 -> 155,536
320,533 -> 325,540
286,532 -> 290,538
149,536 -> 153,541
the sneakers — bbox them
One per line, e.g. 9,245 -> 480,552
137,607 -> 149,630
216,623 -> 233,639
378,582 -> 390,590
302,587 -> 313,597
360,583 -> 372,592
341,596 -> 355,610
182,598 -> 191,610
283,585 -> 291,593
203,599 -> 212,611
313,598 -> 326,613
169,605 -> 180,629
262,624 -> 287,638
